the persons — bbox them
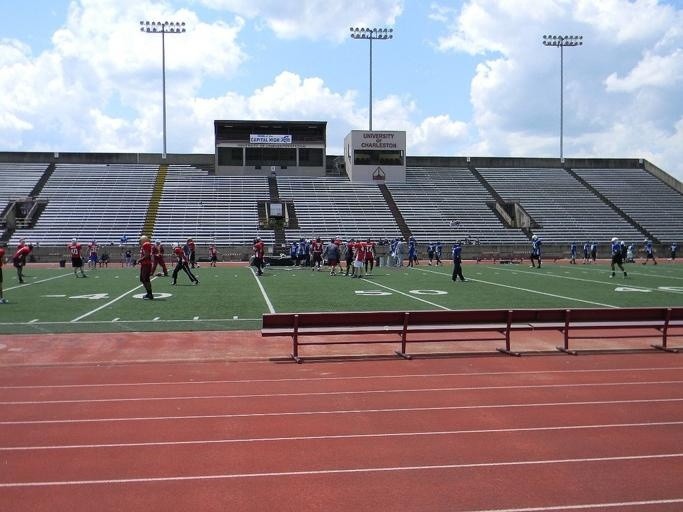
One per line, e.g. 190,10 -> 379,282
670,242 -> 678,260
427,241 -> 443,266
590,241 -> 598,264
248,237 -> 272,276
280,234 -> 420,279
641,240 -> 658,265
582,241 -> 591,264
455,238 -> 481,245
529,235 -> 543,268
619,240 -> 636,263
1,238 -> 34,304
609,237 -> 628,279
170,237 -> 200,286
209,244 -> 222,267
136,235 -> 169,300
451,244 -> 469,282
68,234 -> 133,278
570,241 -> 577,264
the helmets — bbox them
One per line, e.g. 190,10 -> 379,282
531,235 -> 537,241
611,237 -> 618,245
139,236 -> 148,244
171,242 -> 178,249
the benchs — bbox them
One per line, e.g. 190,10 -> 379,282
1,161 -> 682,247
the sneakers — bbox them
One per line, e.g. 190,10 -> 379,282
143,295 -> 152,300
0,298 -> 8,303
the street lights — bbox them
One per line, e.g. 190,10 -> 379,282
539,34 -> 586,165
348,26 -> 396,132
137,20 -> 191,160
